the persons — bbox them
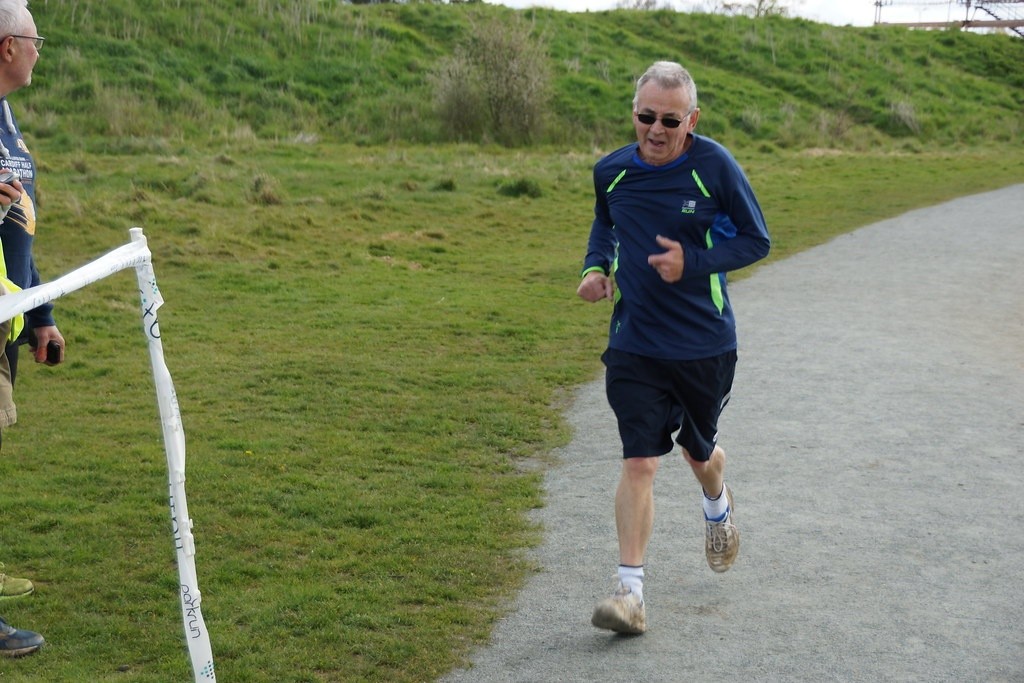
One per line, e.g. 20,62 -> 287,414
0,1 -> 65,656
576,62 -> 772,635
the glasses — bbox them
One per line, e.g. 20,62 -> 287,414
636,107 -> 694,129
0,35 -> 45,50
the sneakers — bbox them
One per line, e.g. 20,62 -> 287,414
0,616 -> 45,656
704,484 -> 740,572
0,560 -> 34,600
590,583 -> 646,634
